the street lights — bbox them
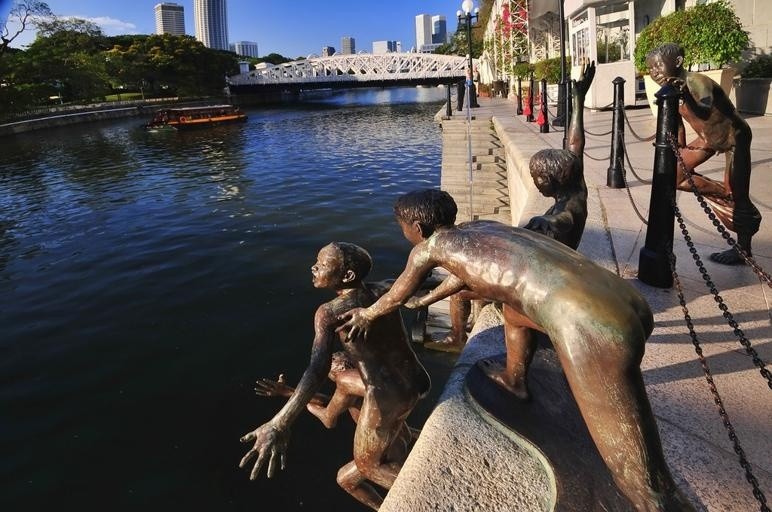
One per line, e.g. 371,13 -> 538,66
455,0 -> 483,112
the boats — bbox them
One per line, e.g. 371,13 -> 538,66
141,104 -> 249,135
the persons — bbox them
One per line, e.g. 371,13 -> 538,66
335,189 -> 697,512
645,43 -> 762,266
239,242 -> 432,490
254,352 -> 412,511
473,67 -> 480,97
424,57 -> 596,353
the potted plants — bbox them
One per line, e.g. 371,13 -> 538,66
635,0 -> 771,117
